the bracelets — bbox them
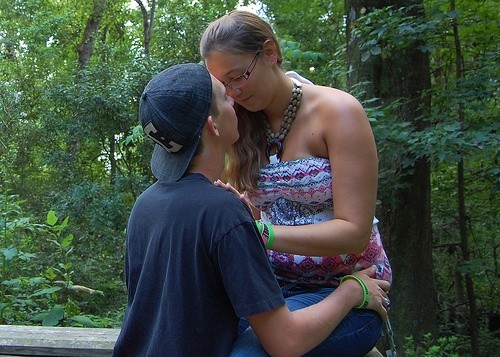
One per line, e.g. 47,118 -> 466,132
255,219 -> 274,250
340,274 -> 369,309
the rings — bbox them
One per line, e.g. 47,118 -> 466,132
384,298 -> 391,309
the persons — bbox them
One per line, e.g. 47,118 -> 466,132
113,62 -> 392,357
200,10 -> 392,357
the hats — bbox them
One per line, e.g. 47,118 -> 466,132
139,61 -> 213,184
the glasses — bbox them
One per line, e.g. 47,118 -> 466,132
223,50 -> 261,88
